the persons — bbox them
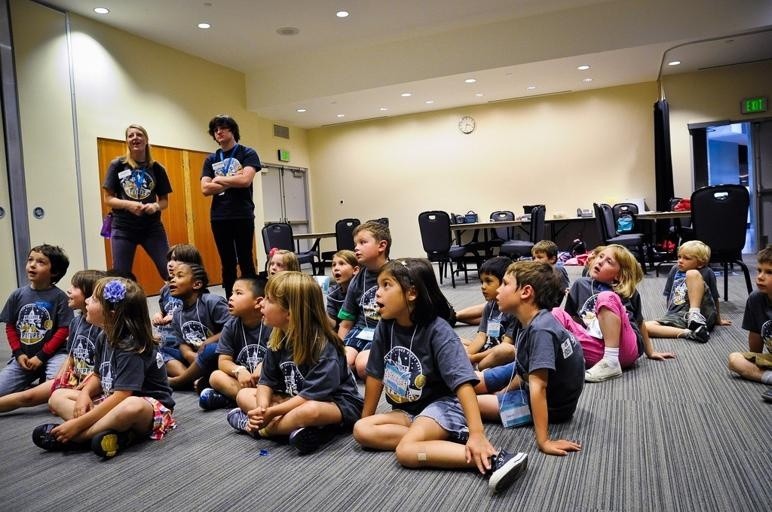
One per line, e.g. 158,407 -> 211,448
467,258 -> 588,458
31,276 -> 177,460
1,271 -> 105,424
352,257 -> 529,491
727,245 -> 772,402
548,244 -> 676,380
103,123 -> 172,283
643,240 -> 733,344
2,243 -> 69,393
457,254 -> 517,371
454,239 -> 570,326
199,115 -> 261,295
154,219 -> 392,454
565,245 -> 604,318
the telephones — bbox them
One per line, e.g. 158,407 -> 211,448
577,208 -> 593,217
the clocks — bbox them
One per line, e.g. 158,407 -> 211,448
459,116 -> 475,133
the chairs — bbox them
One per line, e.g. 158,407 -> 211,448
261,218 -> 390,275
419,210 -> 483,289
522,204 -> 547,243
643,201 -> 650,212
670,198 -> 690,229
500,207 -> 539,261
613,203 -> 640,233
601,204 -> 653,271
451,212 -> 488,277
675,184 -> 752,302
593,203 -> 646,275
484,211 -> 516,256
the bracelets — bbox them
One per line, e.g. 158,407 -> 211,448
154,201 -> 162,211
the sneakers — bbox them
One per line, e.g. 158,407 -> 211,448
585,359 -> 622,382
687,311 -> 710,342
199,389 -> 320,453
33,423 -> 138,459
490,449 -> 528,494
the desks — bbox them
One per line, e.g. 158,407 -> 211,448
449,219 -> 530,246
634,210 -> 692,242
543,216 -> 606,257
